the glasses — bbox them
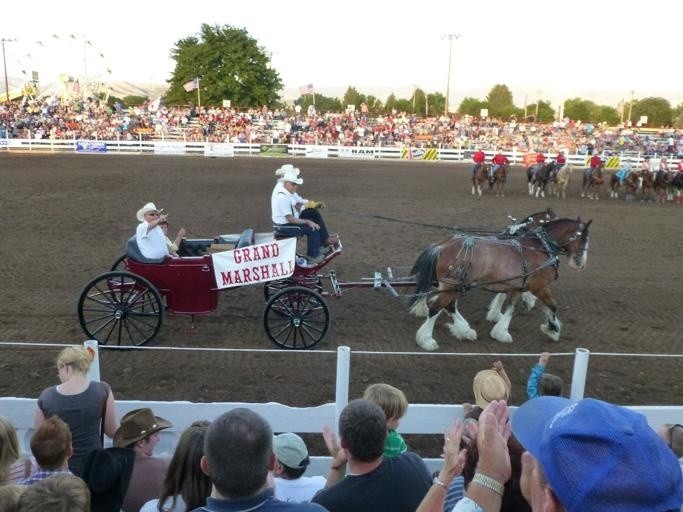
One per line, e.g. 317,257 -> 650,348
149,214 -> 158,216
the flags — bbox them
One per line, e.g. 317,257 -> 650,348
183,77 -> 198,93
299,84 -> 313,96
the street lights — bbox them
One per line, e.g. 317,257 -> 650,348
438,31 -> 461,115
1,36 -> 18,102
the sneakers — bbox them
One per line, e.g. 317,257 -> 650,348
307,234 -> 337,264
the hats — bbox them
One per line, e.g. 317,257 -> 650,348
473,369 -> 683,512
276,164 -> 303,184
113,407 -> 171,448
137,202 -> 157,221
272,433 -> 308,469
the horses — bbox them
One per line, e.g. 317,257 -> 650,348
608,168 -> 683,204
526,160 -> 574,199
401,205 -> 594,352
471,162 -> 511,198
579,160 -> 605,201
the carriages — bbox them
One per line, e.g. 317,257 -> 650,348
76,207 -> 594,351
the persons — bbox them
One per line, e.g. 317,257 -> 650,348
135,202 -> 180,258
156,214 -> 184,257
0,346 -> 680,512
274,164 -> 338,246
270,174 -> 332,267
0,97 -> 683,186
